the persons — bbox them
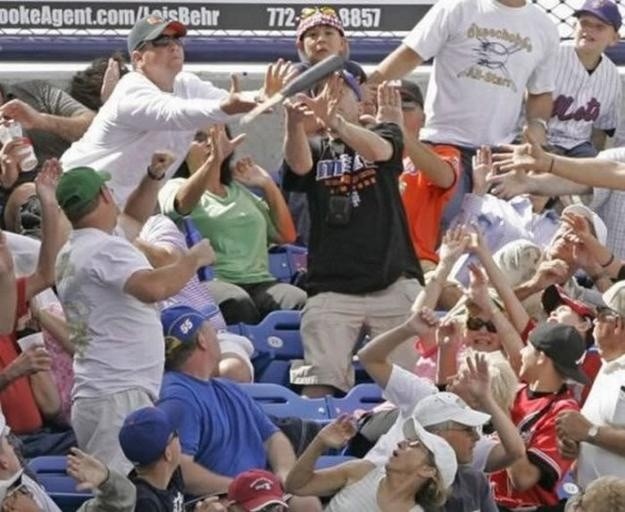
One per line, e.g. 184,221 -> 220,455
0,0 -> 625,512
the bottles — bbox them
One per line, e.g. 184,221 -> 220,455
182,214 -> 213,284
0,111 -> 38,174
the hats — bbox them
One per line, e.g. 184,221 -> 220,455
160,305 -> 220,350
412,392 -> 491,427
563,205 -> 607,246
529,324 -> 593,386
228,469 -> 289,511
571,1 -> 622,32
296,5 -> 344,45
55,167 -> 112,211
583,280 -> 625,314
119,400 -> 186,464
403,416 -> 459,490
0,467 -> 23,504
128,14 -> 186,57
394,78 -> 424,106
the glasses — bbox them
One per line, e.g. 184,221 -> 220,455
4,484 -> 29,497
300,7 -> 334,18
466,317 -> 497,331
151,34 -> 183,46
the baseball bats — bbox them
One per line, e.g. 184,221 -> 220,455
239,53 -> 345,123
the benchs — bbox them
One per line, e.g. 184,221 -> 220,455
18,173 -> 582,504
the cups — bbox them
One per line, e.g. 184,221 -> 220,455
15,331 -> 49,356
